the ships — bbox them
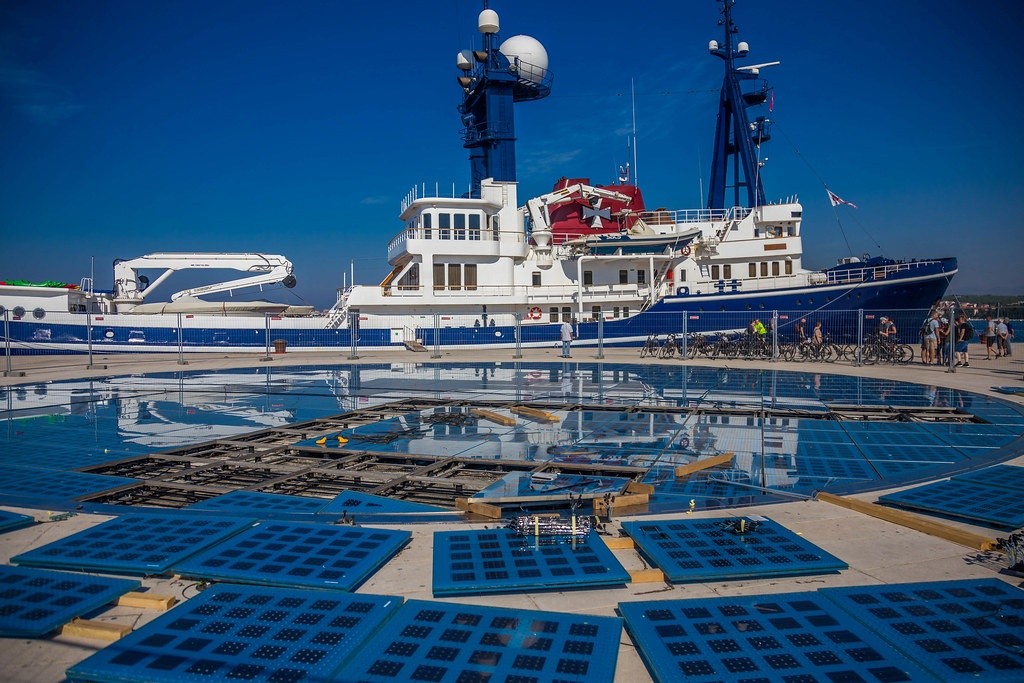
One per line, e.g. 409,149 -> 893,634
1,1 -> 964,356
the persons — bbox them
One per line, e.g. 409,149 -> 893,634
982,315 -> 1014,360
877,315 -> 896,361
799,317 -> 823,357
747,315 -> 779,354
560,319 -> 573,358
920,311 -> 970,367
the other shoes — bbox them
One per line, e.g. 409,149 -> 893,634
955,363 -> 962,367
983,358 -> 991,360
970,326 -> 974,338
962,364 -> 969,367
995,354 -> 1001,359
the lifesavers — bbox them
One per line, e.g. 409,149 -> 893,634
681,245 -> 692,256
530,306 -> 543,320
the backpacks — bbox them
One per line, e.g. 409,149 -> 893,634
920,318 -> 935,335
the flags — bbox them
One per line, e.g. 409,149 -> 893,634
827,189 -> 857,209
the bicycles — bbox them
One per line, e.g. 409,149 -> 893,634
636,328 -> 915,366
